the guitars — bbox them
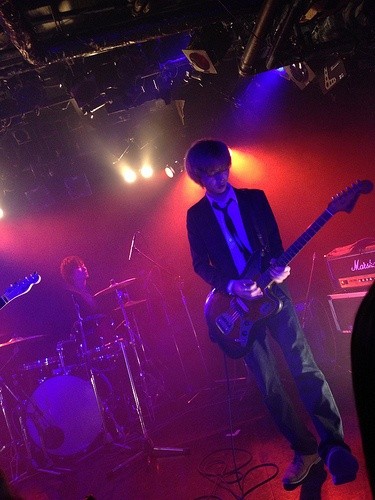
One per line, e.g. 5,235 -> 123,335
203,178 -> 373,360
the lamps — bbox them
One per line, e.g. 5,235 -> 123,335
181,25 -> 235,75
166,156 -> 186,177
263,1 -> 375,97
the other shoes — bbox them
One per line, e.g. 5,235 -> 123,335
325,446 -> 359,486
281,450 -> 322,485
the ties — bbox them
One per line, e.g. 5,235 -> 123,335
212,197 -> 254,264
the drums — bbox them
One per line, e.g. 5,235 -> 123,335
4,314 -> 128,464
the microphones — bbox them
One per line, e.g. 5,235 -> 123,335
128,233 -> 137,260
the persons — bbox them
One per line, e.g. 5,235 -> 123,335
185,139 -> 359,486
58,257 -> 124,339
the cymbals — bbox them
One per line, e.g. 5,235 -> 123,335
111,299 -> 150,312
94,276 -> 136,297
0,333 -> 48,350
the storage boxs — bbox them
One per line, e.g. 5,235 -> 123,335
327,289 -> 368,333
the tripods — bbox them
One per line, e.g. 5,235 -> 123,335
0,245 -> 247,487
